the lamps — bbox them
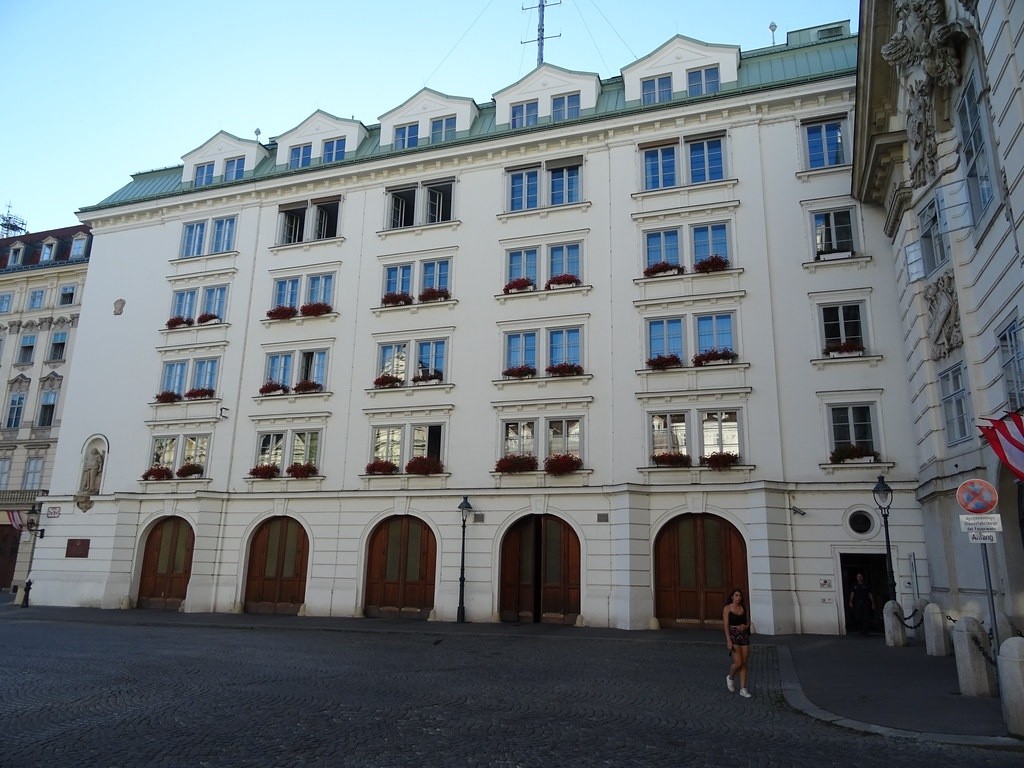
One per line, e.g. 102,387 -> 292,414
26,505 -> 45,539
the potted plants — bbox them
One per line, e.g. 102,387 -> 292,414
501,363 -> 536,380
176,464 -> 205,479
645,352 -> 683,371
417,286 -> 450,303
292,379 -> 323,395
503,275 -> 536,294
693,254 -> 731,275
285,462 -> 319,479
165,315 -> 194,329
829,445 -> 880,464
259,381 -> 289,396
692,347 -> 738,367
647,450 -> 693,470
265,305 -> 298,320
544,273 -> 581,291
365,460 -> 398,477
185,386 -> 216,401
373,373 -> 405,388
697,451 -> 743,472
381,290 -> 414,308
815,248 -> 856,260
299,302 -> 333,316
247,462 -> 281,479
545,362 -> 585,378
196,312 -> 221,325
644,260 -> 686,278
410,373 -> 440,386
543,451 -> 582,478
153,390 -> 183,404
822,341 -> 865,357
141,466 -> 173,481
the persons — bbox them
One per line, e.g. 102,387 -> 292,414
849,572 -> 875,632
84,448 -> 102,492
723,589 -> 752,697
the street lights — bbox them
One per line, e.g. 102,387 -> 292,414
453,496 -> 472,623
872,475 -> 898,601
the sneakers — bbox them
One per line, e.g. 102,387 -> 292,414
726,675 -> 735,692
740,687 -> 751,697
